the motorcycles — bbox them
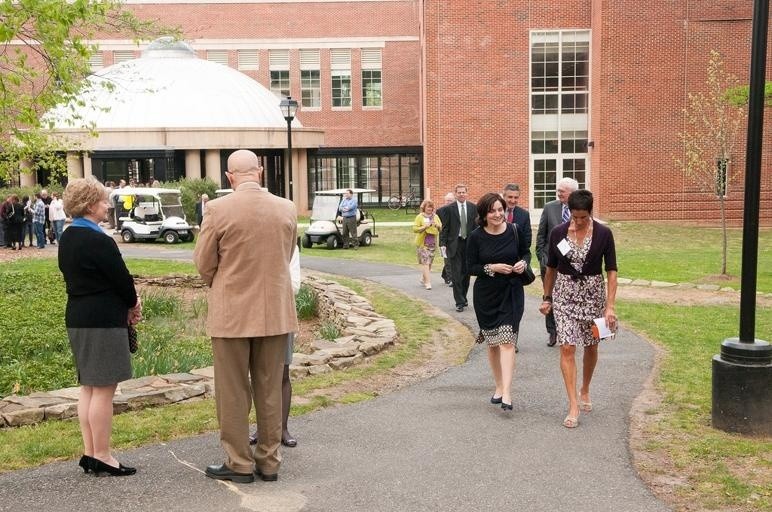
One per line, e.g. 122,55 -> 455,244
111,187 -> 198,244
303,189 -> 378,247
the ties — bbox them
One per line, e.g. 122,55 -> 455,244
461,204 -> 468,240
562,206 -> 569,224
506,209 -> 513,224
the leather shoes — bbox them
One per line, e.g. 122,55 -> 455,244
456,306 -> 463,312
465,301 -> 468,306
256,468 -> 277,482
548,334 -> 556,346
206,462 -> 254,483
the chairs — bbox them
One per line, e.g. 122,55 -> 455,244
135,206 -> 145,220
355,209 -> 362,224
144,207 -> 159,221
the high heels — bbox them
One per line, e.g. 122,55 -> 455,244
491,393 -> 502,403
563,409 -> 580,427
425,283 -> 431,289
88,457 -> 136,477
79,453 -> 91,472
580,389 -> 592,411
502,402 -> 513,412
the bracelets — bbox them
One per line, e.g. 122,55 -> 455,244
542,294 -> 550,301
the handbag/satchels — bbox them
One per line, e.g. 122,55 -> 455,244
425,223 -> 439,234
511,225 -> 536,286
592,317 -> 618,341
128,324 -> 139,353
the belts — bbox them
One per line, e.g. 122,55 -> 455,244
344,215 -> 355,218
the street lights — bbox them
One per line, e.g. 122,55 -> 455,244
280,95 -> 299,202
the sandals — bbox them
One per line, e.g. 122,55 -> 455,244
282,436 -> 298,449
250,433 -> 258,445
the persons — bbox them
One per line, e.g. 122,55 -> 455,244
0,174 -> 163,249
502,185 -> 531,354
536,177 -> 579,347
439,185 -> 477,312
247,244 -> 301,451
195,193 -> 209,228
339,190 -> 359,250
412,199 -> 442,289
466,193 -> 535,411
194,148 -> 298,484
58,176 -> 143,475
538,190 -> 618,428
436,192 -> 456,286
